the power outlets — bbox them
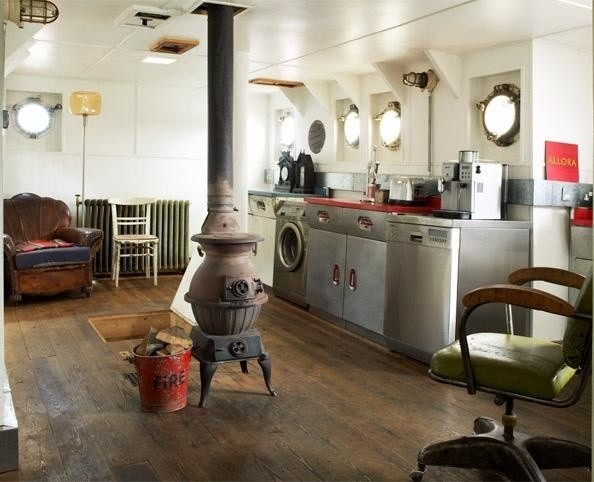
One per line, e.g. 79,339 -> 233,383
561,186 -> 573,200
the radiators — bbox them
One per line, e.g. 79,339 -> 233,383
75,195 -> 192,277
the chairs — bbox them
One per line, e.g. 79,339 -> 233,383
408,262 -> 593,482
108,196 -> 160,288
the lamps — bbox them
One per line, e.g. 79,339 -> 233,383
70,91 -> 102,227
0,1 -> 60,351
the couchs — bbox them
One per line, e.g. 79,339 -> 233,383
4,192 -> 105,307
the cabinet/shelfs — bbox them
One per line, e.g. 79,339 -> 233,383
306,203 -> 386,346
247,192 -> 324,312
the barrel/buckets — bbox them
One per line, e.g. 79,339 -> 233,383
132,342 -> 195,413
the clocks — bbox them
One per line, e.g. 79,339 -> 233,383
291,148 -> 316,194
275,151 -> 294,191
